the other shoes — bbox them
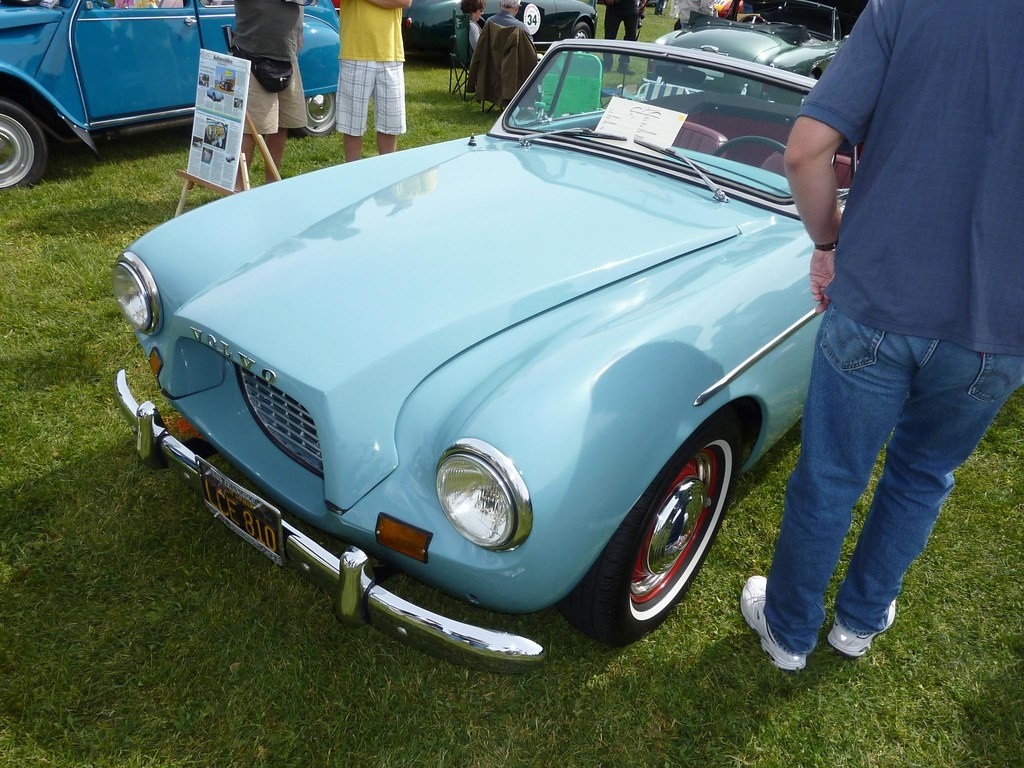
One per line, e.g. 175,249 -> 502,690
603,64 -> 611,73
617,63 -> 636,74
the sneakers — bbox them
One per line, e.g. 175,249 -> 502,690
740,576 -> 806,670
828,600 -> 896,657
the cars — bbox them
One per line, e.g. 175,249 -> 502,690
0,0 -> 342,190
112,37 -> 860,674
646,0 -> 858,111
404,0 -> 599,64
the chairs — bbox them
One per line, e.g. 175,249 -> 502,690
600,88 -> 622,108
763,150 -> 859,189
672,121 -> 728,159
450,9 -> 603,119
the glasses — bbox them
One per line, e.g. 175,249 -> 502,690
480,9 -> 483,13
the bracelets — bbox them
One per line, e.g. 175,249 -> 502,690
814,241 -> 838,251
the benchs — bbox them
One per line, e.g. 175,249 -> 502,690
758,7 -> 857,39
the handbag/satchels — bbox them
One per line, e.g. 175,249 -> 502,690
232,48 -> 294,92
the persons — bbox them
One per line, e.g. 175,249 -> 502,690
333,0 -> 412,162
230,0 -> 308,192
654,0 -> 664,15
466,0 -> 537,107
674,0 -> 715,30
458,0 -> 486,69
739,0 -> 1024,673
600,0 -> 648,75
